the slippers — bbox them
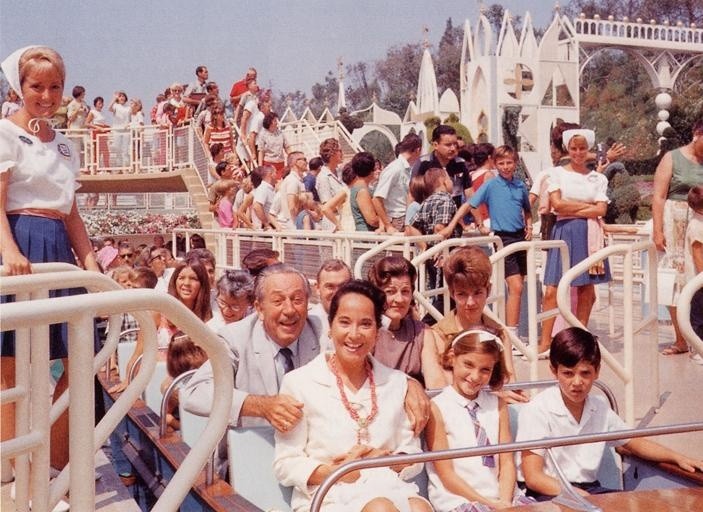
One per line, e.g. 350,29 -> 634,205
660,343 -> 689,356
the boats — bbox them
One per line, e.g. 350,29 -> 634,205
90,248 -> 702,512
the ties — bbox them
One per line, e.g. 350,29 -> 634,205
280,347 -> 296,371
467,403 -> 496,469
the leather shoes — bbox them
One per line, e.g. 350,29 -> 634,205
521,349 -> 552,361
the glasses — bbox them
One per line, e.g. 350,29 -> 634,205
120,253 -> 134,258
335,149 -> 344,153
148,254 -> 161,262
214,296 -> 251,312
296,157 -> 307,162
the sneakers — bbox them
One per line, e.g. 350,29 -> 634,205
509,341 -> 524,358
